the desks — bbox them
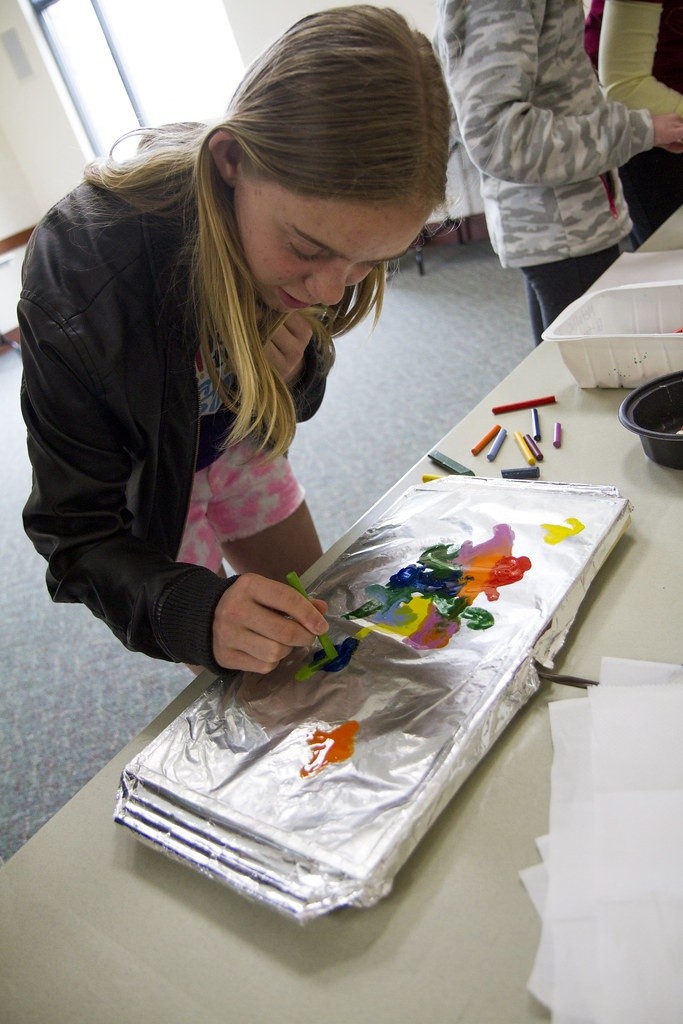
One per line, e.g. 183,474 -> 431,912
1,205 -> 683,1023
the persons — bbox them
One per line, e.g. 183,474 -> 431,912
434,0 -> 683,345
14,0 -> 453,672
